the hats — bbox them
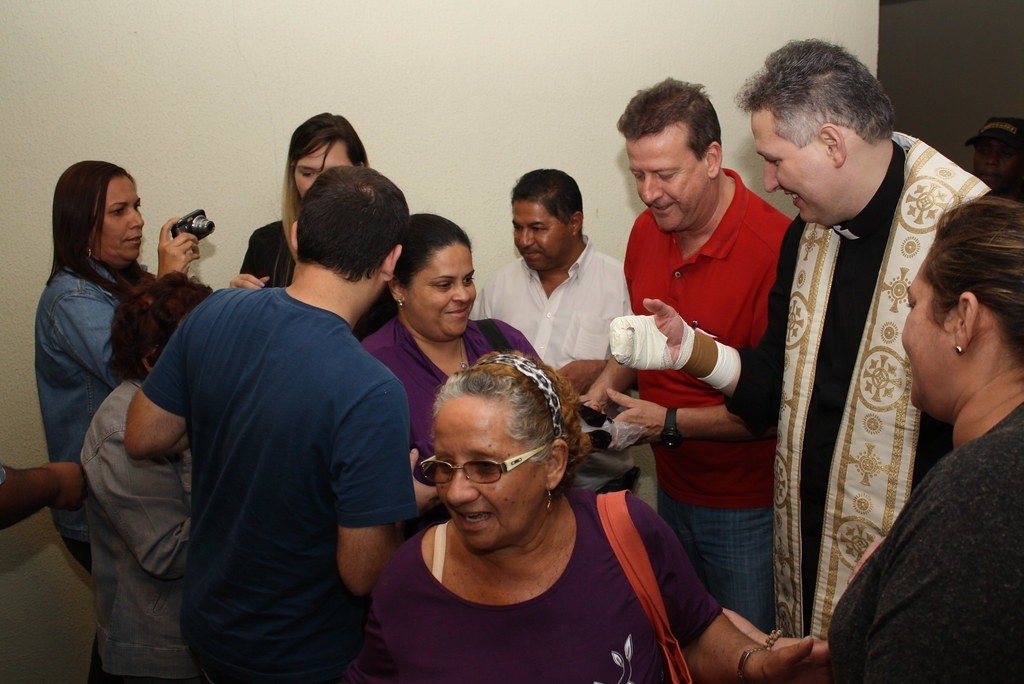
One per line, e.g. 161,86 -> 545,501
965,117 -> 1024,149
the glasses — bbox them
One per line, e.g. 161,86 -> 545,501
419,440 -> 553,483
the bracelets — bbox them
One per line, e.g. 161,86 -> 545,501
737,648 -> 765,684
763,629 -> 782,651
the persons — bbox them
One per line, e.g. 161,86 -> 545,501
965,117 -> 1024,202
610,40 -> 993,640
79,272 -> 214,684
468,168 -> 634,491
578,78 -> 793,635
337,350 -> 812,684
360,213 -> 606,541
228,113 -> 398,341
125,166 -> 442,684
34,160 -> 200,575
0,462 -> 86,528
723,195 -> 1024,684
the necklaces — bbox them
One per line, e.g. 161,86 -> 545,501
457,339 -> 467,369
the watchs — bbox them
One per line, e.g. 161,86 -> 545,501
660,408 -> 682,449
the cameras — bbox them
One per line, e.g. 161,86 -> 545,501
170,209 -> 215,242
